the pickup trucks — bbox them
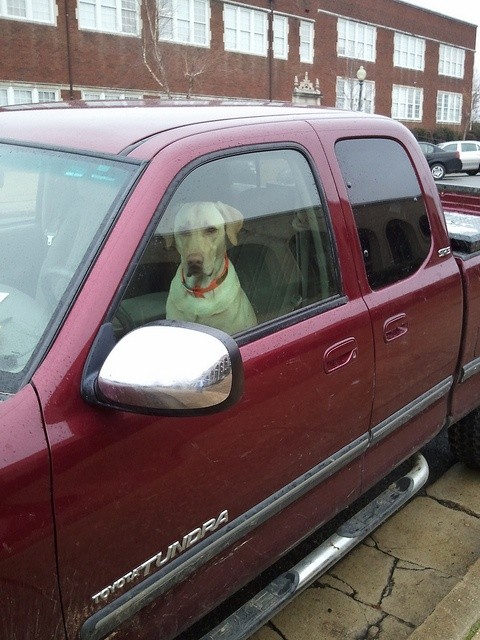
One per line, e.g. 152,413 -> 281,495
0,105 -> 480,639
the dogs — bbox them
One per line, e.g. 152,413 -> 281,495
156,200 -> 259,337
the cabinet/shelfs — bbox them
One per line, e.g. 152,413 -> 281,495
229,188 -> 301,324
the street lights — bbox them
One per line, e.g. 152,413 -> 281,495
357,65 -> 366,110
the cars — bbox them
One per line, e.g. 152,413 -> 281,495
436,140 -> 480,175
417,141 -> 462,180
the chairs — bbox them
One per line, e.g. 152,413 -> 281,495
37,176 -> 120,311
